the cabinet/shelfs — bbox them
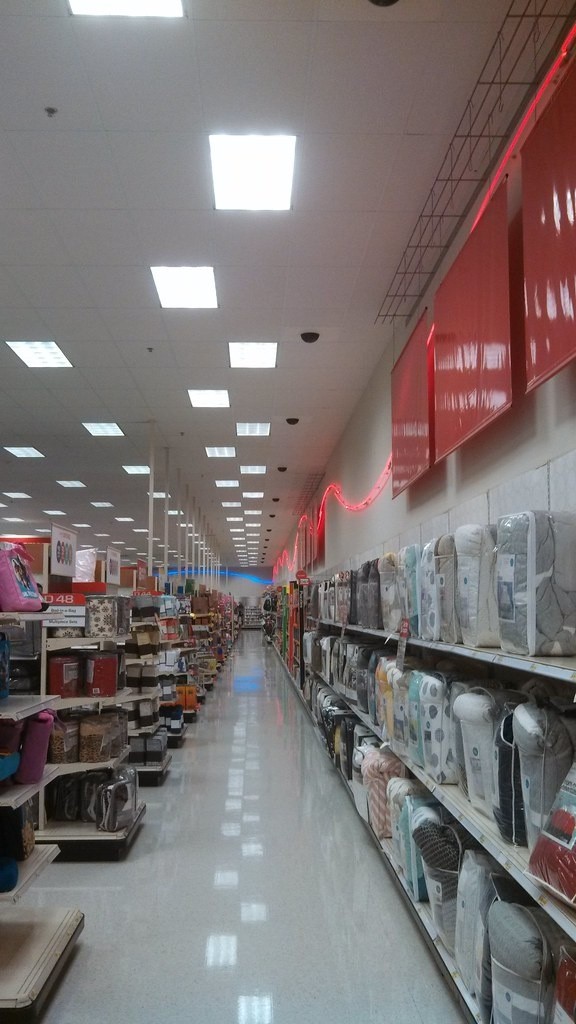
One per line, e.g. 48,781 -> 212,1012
41,635 -> 147,862
125,623 -> 172,787
306,615 -> 576,1024
0,611 -> 85,1024
171,578 -> 310,727
